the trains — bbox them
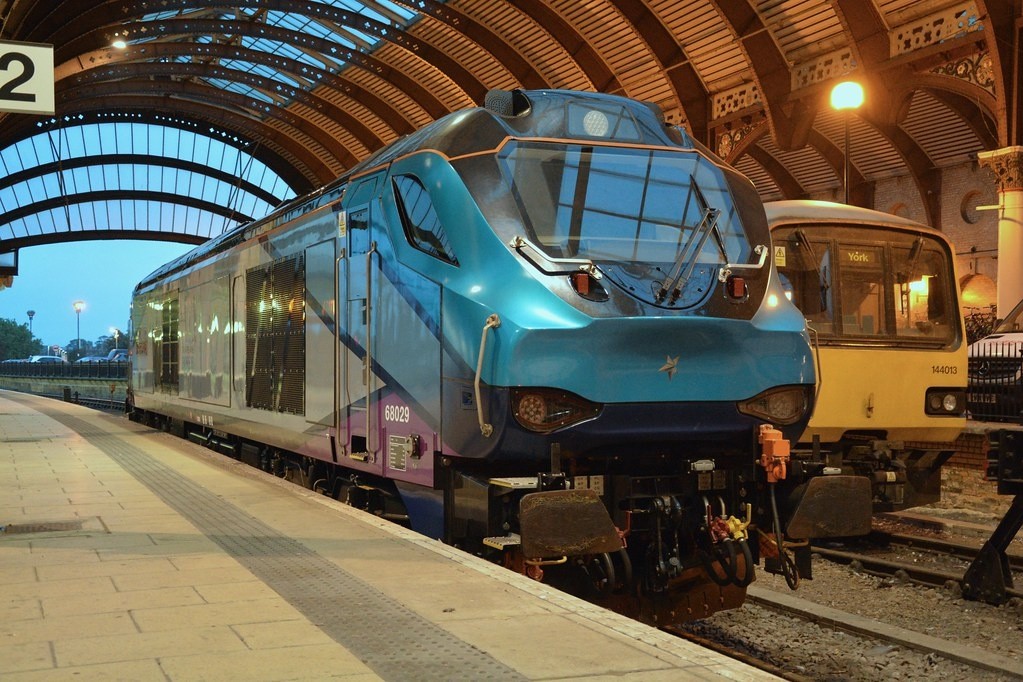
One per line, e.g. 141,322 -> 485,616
125,86 -> 823,606
758,198 -> 971,553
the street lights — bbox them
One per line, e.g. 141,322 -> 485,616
26,310 -> 35,332
828,81 -> 867,205
72,299 -> 85,360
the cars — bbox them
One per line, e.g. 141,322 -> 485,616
107,349 -> 127,363
960,297 -> 1023,423
1,355 -> 70,364
74,356 -> 107,363
